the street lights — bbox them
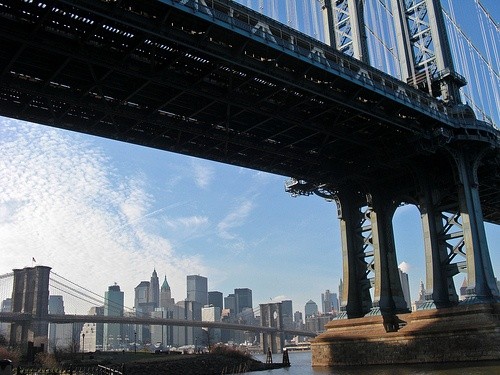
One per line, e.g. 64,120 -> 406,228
134,332 -> 137,353
82,333 -> 85,360
